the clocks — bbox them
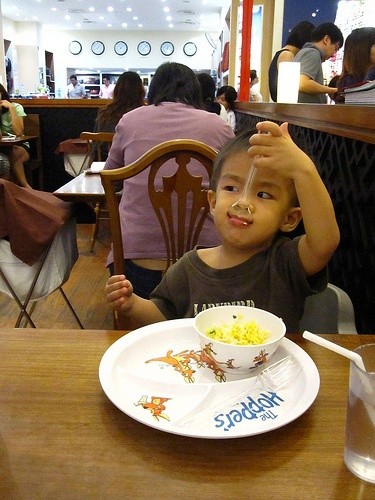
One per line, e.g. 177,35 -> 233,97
160,41 -> 173,56
113,41 -> 127,55
69,41 -> 82,56
182,42 -> 196,56
92,41 -> 105,54
137,41 -> 151,56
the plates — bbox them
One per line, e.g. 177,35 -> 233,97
98,318 -> 320,439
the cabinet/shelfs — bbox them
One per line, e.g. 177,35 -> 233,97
75,72 -> 123,97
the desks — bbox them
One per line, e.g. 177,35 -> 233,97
0,327 -> 375,500
53,170 -> 129,220
0,134 -> 39,184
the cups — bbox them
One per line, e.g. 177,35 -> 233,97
277,62 -> 301,104
344,343 -> 375,485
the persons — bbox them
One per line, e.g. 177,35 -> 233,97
104,61 -> 235,299
99,75 -> 115,99
269,20 -> 316,104
328,75 -> 340,101
215,86 -> 238,134
249,70 -> 263,101
0,83 -> 34,190
106,120 -> 342,333
293,22 -> 344,103
92,70 -> 145,162
196,73 -> 229,123
65,74 -> 86,99
335,27 -> 375,105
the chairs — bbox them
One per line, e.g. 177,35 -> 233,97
299,281 -> 359,336
79,126 -> 220,326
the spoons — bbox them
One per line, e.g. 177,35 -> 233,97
228,130 -> 270,224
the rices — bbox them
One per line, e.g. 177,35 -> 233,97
206,316 -> 270,345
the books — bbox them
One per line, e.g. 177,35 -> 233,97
339,79 -> 375,104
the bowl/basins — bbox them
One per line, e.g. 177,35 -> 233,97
194,305 -> 286,370
